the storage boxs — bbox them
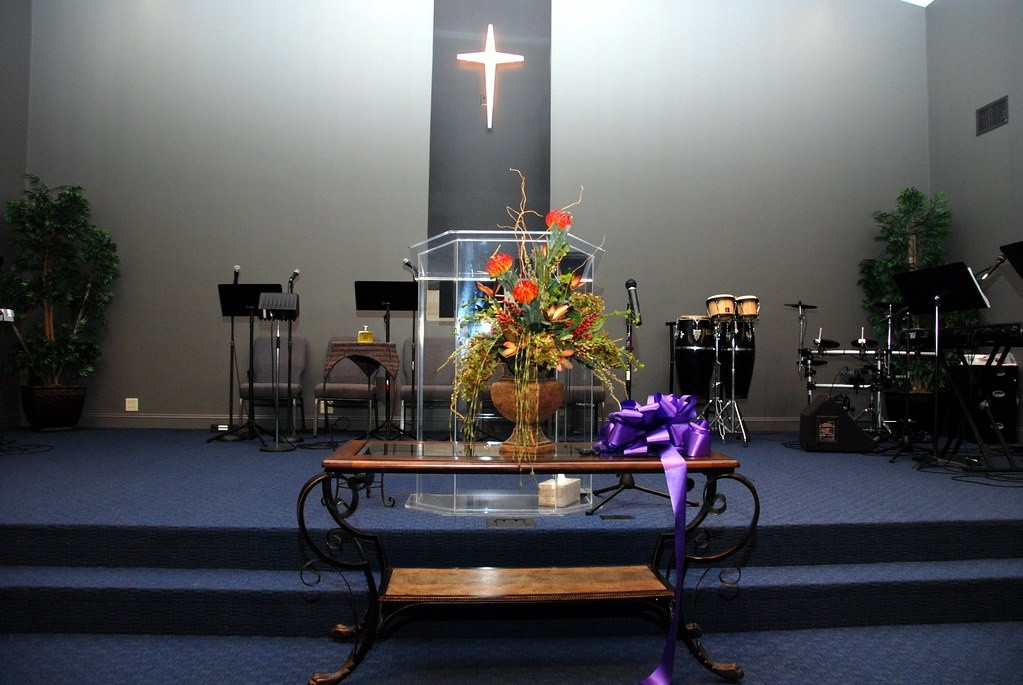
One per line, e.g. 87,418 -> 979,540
538,478 -> 581,507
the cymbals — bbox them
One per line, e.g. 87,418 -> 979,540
812,339 -> 840,348
784,303 -> 818,309
850,338 -> 879,349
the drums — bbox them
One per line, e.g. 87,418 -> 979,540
736,296 -> 760,319
673,314 -> 756,399
706,295 -> 737,320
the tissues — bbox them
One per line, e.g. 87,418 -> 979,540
538,473 -> 581,507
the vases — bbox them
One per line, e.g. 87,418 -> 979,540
491,376 -> 565,455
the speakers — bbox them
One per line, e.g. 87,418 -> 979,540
799,394 -> 877,454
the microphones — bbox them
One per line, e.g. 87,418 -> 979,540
625,279 -> 643,327
982,254 -> 1007,282
234,265 -> 241,284
403,258 -> 420,273
289,269 -> 301,282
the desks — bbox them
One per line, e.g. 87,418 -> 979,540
298,440 -> 759,683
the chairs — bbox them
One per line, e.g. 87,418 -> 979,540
238,334 -> 307,439
398,335 -> 457,436
557,357 -> 606,435
314,336 -> 382,439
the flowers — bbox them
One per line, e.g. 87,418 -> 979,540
433,170 -> 642,449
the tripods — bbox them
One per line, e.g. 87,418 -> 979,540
206,284 -> 283,448
354,280 -> 417,442
583,305 -> 700,517
696,320 -> 752,448
843,305 -> 935,463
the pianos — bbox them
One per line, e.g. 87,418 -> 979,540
898,319 -> 1022,471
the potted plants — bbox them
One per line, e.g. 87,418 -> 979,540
0,172 -> 120,432
855,185 -> 978,431
